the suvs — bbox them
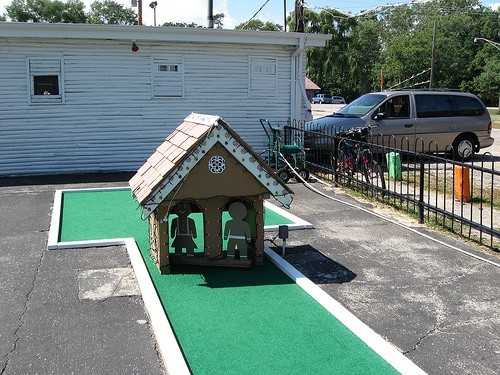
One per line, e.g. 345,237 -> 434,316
311,93 -> 332,105
305,91 -> 494,166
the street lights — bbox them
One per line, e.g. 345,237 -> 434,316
149,0 -> 158,27
130,0 -> 143,25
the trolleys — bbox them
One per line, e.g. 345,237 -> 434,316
259,118 -> 310,184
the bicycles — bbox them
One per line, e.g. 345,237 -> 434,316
329,123 -> 387,197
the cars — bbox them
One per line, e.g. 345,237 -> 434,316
331,96 -> 345,104
